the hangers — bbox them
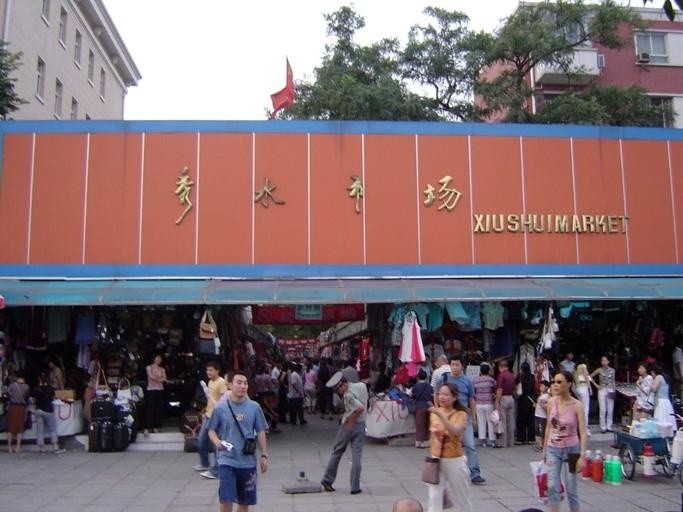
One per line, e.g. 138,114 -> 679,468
404,304 -> 418,319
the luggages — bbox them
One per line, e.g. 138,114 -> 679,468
88,418 -> 137,452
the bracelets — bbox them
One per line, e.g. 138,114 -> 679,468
260,454 -> 268,458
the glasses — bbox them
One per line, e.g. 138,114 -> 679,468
552,380 -> 567,384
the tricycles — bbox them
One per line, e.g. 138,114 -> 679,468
609,412 -> 683,481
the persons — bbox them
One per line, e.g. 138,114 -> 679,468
433,354 -> 487,485
198,368 -> 251,480
46,359 -> 67,392
253,354 -> 390,434
411,344 -> 682,451
28,371 -> 66,454
392,497 -> 423,512
5,368 -> 31,455
141,351 -> 167,437
420,382 -> 474,512
205,369 -> 270,512
320,370 -> 368,495
542,370 -> 588,512
191,361 -> 227,473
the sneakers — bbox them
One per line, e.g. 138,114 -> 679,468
39,447 -> 66,455
514,439 -> 536,446
320,480 -> 335,492
192,462 -> 216,479
480,441 -> 497,449
470,475 -> 486,483
535,446 -> 544,452
350,488 -> 361,495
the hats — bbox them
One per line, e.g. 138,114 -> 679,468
324,370 -> 345,389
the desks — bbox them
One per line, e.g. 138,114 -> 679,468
363,392 -> 420,444
0,394 -> 87,441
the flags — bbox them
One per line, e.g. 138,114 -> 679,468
268,59 -> 296,120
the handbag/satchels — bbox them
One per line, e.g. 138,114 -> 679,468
607,391 -> 617,400
87,305 -> 224,420
240,438 -> 256,456
420,455 -> 440,485
529,460 -> 565,504
184,427 -> 218,453
566,452 -> 581,474
493,407 -> 504,435
